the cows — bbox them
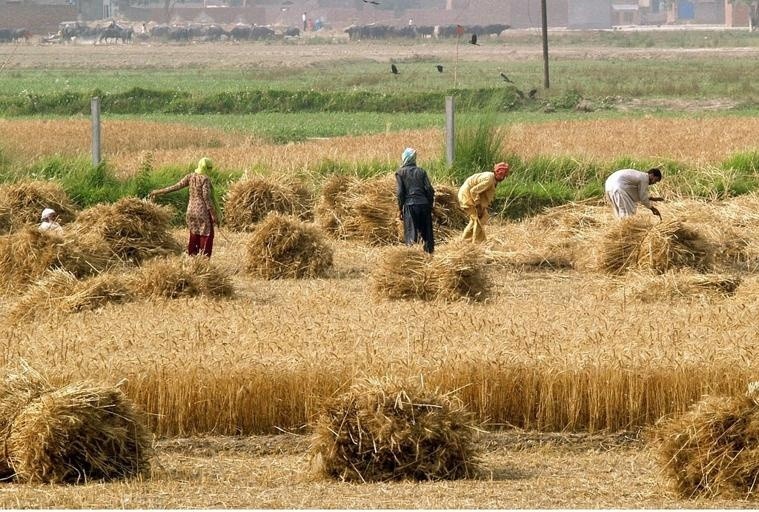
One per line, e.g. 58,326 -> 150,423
0,29 -> 30,44
61,22 -> 300,44
343,23 -> 511,41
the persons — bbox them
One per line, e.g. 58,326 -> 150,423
37,206 -> 64,233
604,167 -> 667,225
146,156 -> 219,257
302,11 -> 323,31
394,146 -> 437,255
457,161 -> 512,242
